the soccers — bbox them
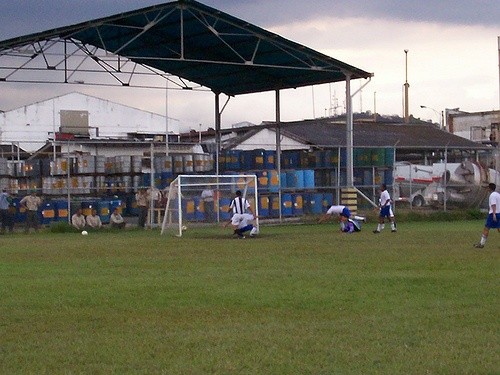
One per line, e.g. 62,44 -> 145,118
82,231 -> 88,236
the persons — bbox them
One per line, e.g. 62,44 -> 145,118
224,213 -> 257,240
146,182 -> 161,228
135,185 -> 149,230
0,187 -> 11,236
472,183 -> 500,249
19,188 -> 43,234
162,175 -> 186,229
373,182 -> 397,234
71,208 -> 88,232
318,204 -> 367,232
201,184 -> 216,222
110,208 -> 126,231
228,189 -> 255,238
86,208 -> 103,230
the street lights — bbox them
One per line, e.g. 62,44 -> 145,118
420,105 -> 443,130
402,48 -> 411,124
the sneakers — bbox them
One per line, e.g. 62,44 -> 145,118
474,243 -> 484,248
372,227 -> 397,233
